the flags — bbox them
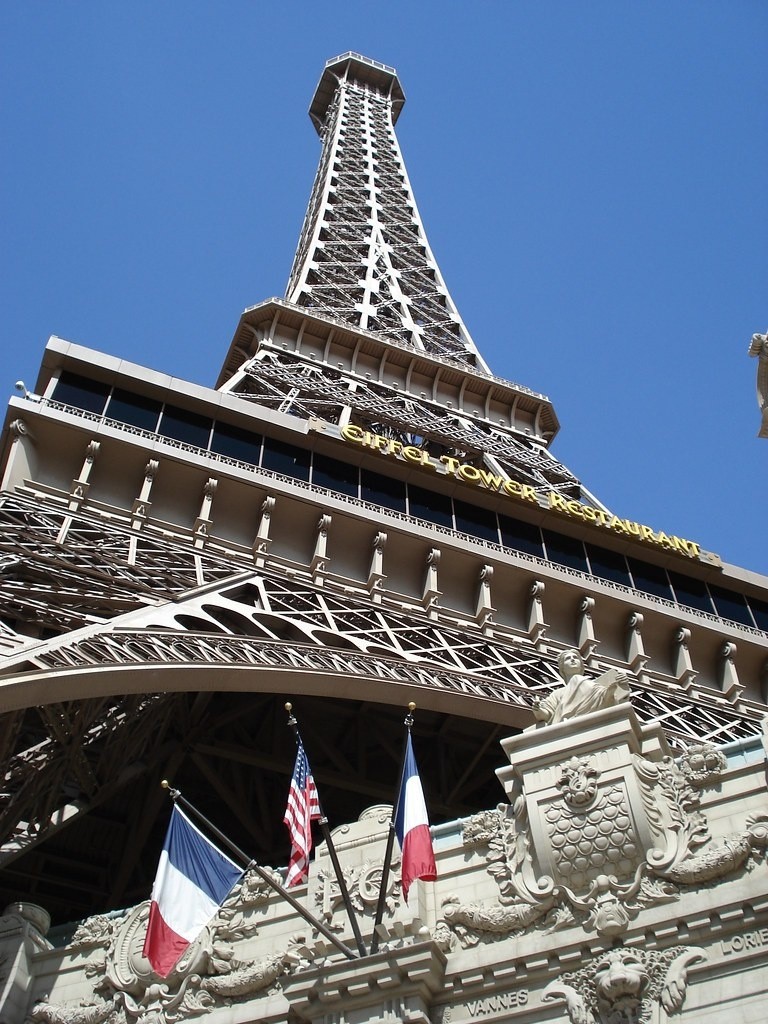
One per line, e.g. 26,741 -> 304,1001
392,727 -> 438,907
281,739 -> 330,893
141,801 -> 247,981
